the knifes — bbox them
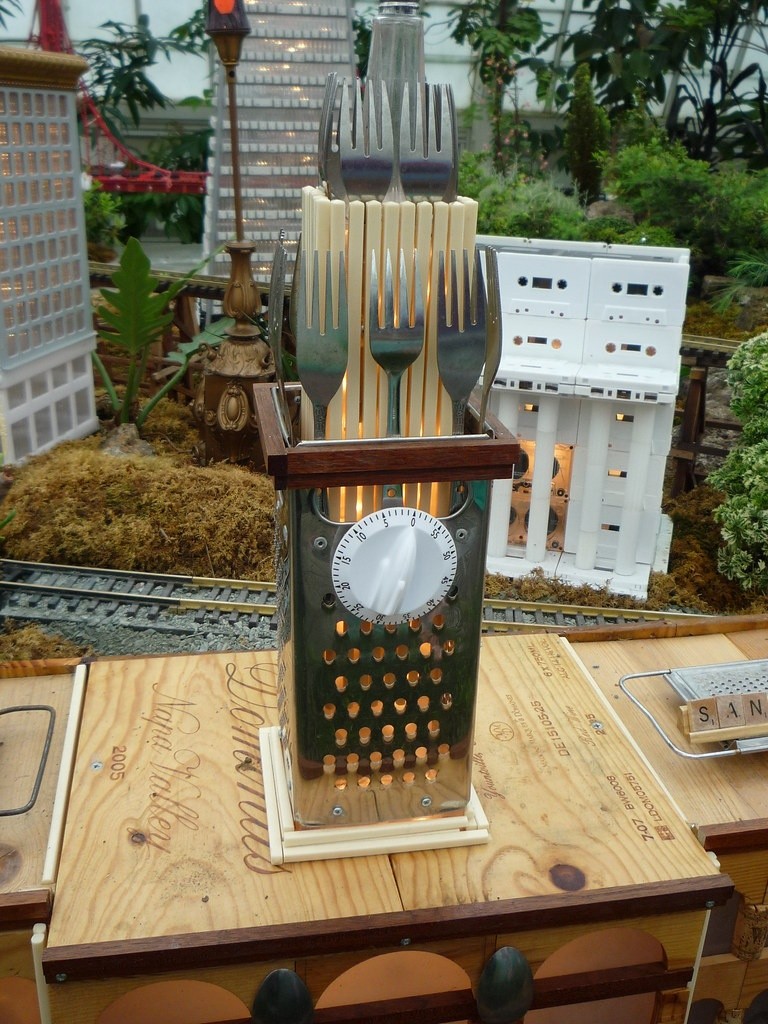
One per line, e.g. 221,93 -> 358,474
426,78 -> 458,202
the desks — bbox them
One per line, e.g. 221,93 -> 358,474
0,632 -> 768,1024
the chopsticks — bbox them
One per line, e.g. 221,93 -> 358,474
296,181 -> 481,525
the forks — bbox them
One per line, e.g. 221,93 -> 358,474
479,242 -> 504,433
400,81 -> 449,202
316,71 -> 339,197
267,225 -> 293,441
296,248 -> 349,442
368,248 -> 425,436
437,247 -> 486,435
341,76 -> 393,202
